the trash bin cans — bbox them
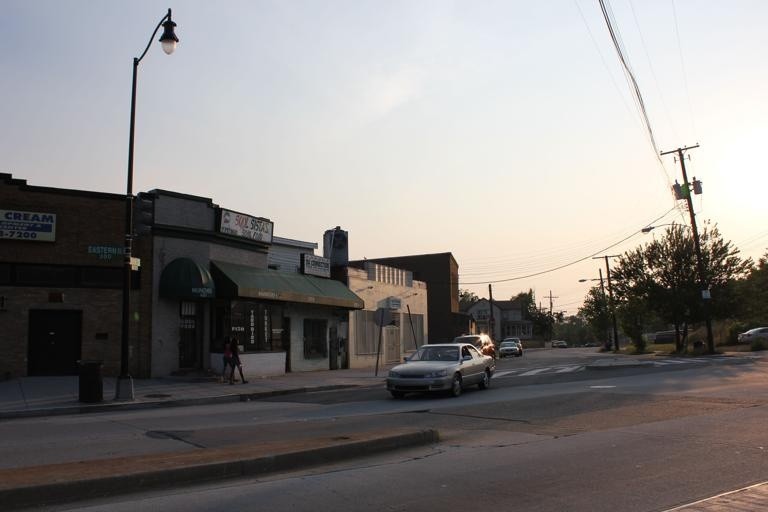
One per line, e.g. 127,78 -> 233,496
78,360 -> 104,402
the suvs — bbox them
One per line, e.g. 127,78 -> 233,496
453,332 -> 497,365
502,337 -> 522,354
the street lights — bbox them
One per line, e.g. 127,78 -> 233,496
642,221 -> 715,351
114,3 -> 191,402
578,268 -> 609,351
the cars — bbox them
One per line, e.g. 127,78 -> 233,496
737,327 -> 768,347
496,342 -> 521,358
584,340 -> 602,347
551,338 -> 569,348
385,342 -> 494,396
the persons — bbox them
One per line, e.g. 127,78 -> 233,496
229,337 -> 248,384
221,335 -> 241,382
